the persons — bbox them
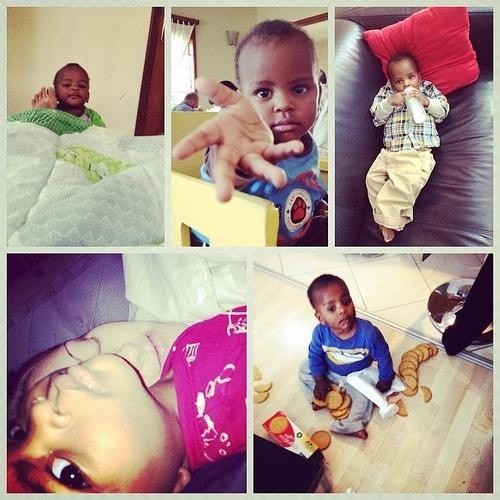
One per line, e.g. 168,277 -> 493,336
174,17 -> 329,248
173,92 -> 203,111
7,304 -> 246,493
295,274 -> 396,439
31,87 -> 58,109
5,321 -> 190,419
365,52 -> 451,242
55,63 -> 107,127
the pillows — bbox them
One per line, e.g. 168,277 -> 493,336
360,8 -> 481,96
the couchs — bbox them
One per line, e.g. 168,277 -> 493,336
336,8 -> 494,244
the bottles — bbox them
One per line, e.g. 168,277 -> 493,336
403,86 -> 428,125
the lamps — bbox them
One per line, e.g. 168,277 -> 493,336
224,29 -> 239,48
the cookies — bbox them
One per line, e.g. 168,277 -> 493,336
252,342 -> 438,451
